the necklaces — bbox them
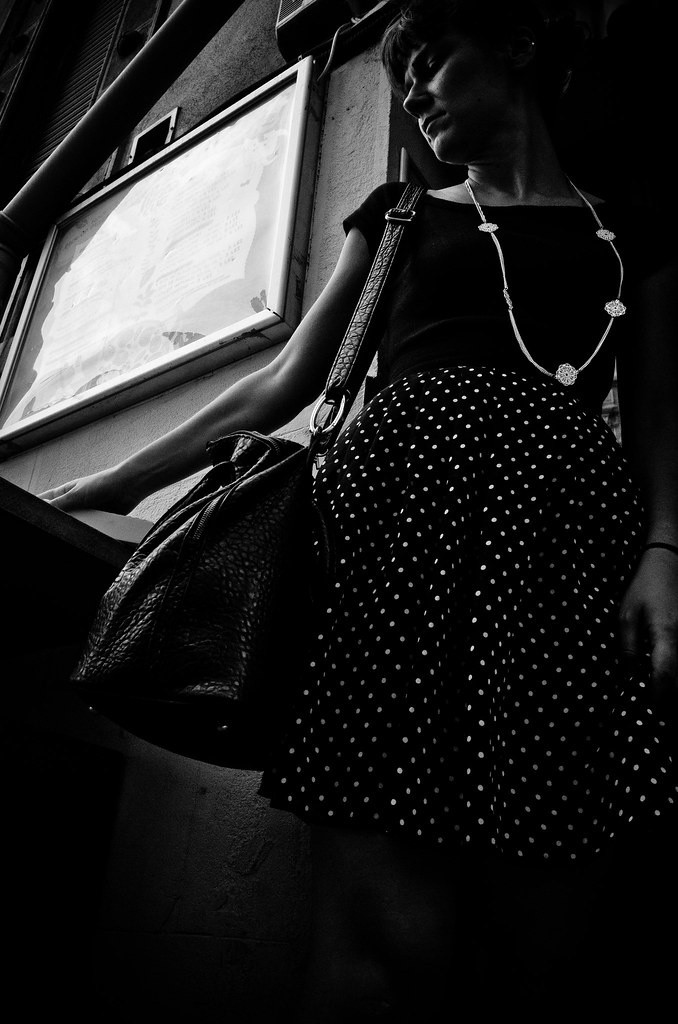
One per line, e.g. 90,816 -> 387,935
464,172 -> 627,387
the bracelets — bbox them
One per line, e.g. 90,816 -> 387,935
641,542 -> 678,555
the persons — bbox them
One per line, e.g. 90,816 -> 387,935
36,0 -> 678,1024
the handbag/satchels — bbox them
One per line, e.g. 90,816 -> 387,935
71,428 -> 313,750
